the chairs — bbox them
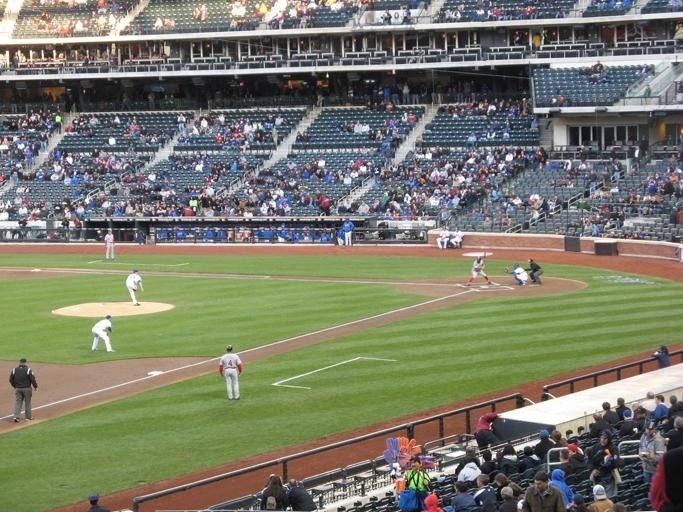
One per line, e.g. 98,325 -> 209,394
1,0 -> 683,243
334,422 -> 667,512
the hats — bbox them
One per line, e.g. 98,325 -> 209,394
624,409 -> 631,417
593,484 -> 607,500
568,443 -> 580,454
644,421 -> 656,430
227,345 -> 232,350
105,315 -> 112,318
540,429 -> 549,439
600,431 -> 608,438
133,270 -> 140,273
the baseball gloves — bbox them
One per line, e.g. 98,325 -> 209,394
504,267 -> 509,273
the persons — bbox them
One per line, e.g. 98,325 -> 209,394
524,258 -> 544,285
220,345 -> 243,400
505,263 -> 531,285
9,358 -> 38,423
0,0 -> 682,249
104,229 -> 116,262
91,315 -> 114,352
126,269 -> 144,306
466,256 -> 492,285
256,473 -> 317,510
89,493 -> 104,511
652,346 -> 671,369
397,390 -> 681,510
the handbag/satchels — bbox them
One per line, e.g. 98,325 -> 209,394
398,492 -> 429,512
